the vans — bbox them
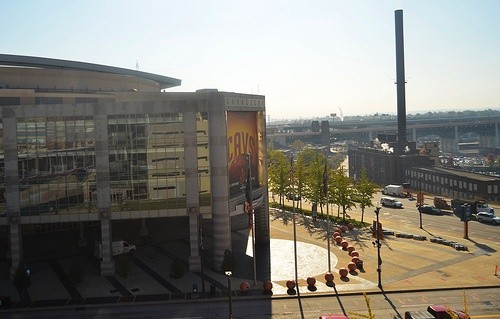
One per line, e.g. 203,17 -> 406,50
381,197 -> 403,207
93,237 -> 137,259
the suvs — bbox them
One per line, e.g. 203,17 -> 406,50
476,211 -> 500,224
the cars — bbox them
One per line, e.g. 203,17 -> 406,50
418,204 -> 440,214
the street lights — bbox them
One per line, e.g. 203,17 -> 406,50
21,266 -> 30,304
371,206 -> 385,288
225,268 -> 234,319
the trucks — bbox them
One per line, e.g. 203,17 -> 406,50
382,185 -> 403,195
404,305 -> 468,319
450,198 -> 494,216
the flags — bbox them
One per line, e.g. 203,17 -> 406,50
246,157 -> 253,235
289,156 -> 294,177
324,163 -> 328,198
